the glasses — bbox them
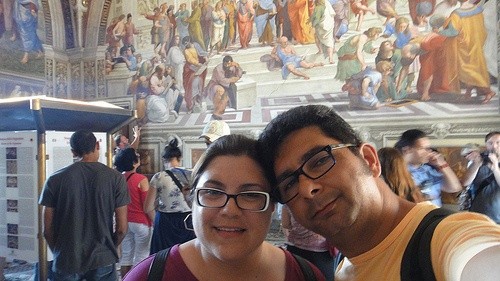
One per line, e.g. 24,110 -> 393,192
193,187 -> 274,213
270,143 -> 359,206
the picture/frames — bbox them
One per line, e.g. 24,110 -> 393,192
137,139 -> 164,176
182,139 -> 210,169
383,133 -> 487,204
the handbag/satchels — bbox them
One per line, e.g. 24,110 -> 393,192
456,173 -> 496,212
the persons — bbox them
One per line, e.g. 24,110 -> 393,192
112,125 -> 141,175
255,105 -> 500,281
114,146 -> 152,280
121,134 -> 329,281
37,129 -> 131,281
144,138 -> 197,256
281,204 -> 335,281
375,147 -> 419,204
393,129 -> 462,210
459,132 -> 500,225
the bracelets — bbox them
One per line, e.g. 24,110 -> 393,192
437,163 -> 450,171
